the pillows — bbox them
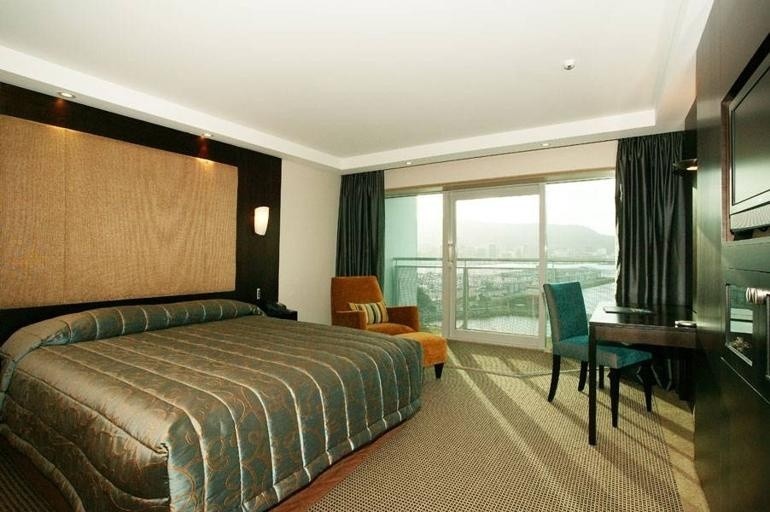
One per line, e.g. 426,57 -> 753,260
348,299 -> 391,324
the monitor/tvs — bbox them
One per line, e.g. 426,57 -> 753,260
728,53 -> 770,234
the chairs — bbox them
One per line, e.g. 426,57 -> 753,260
331,275 -> 419,336
540,280 -> 653,429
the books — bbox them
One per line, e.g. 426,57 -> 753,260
604,307 -> 655,316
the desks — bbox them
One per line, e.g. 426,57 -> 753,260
583,299 -> 698,445
265,307 -> 298,321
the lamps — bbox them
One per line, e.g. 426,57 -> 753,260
252,204 -> 270,238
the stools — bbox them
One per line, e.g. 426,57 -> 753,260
395,333 -> 448,379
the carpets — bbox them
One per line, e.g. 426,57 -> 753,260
266,340 -> 681,512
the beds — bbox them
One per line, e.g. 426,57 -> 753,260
0,296 -> 424,512
